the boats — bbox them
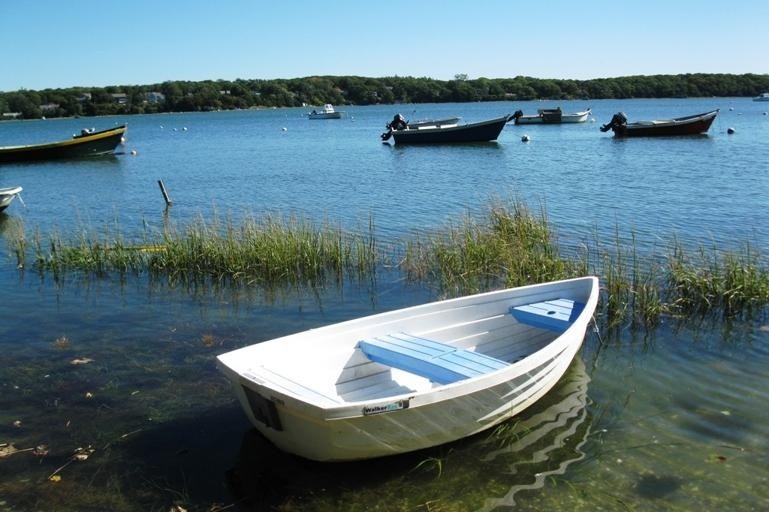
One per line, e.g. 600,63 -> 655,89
506,107 -> 592,124
381,112 -> 510,144
0,186 -> 23,213
599,109 -> 721,136
0,125 -> 126,164
214,275 -> 600,462
753,93 -> 769,101
306,104 -> 346,119
